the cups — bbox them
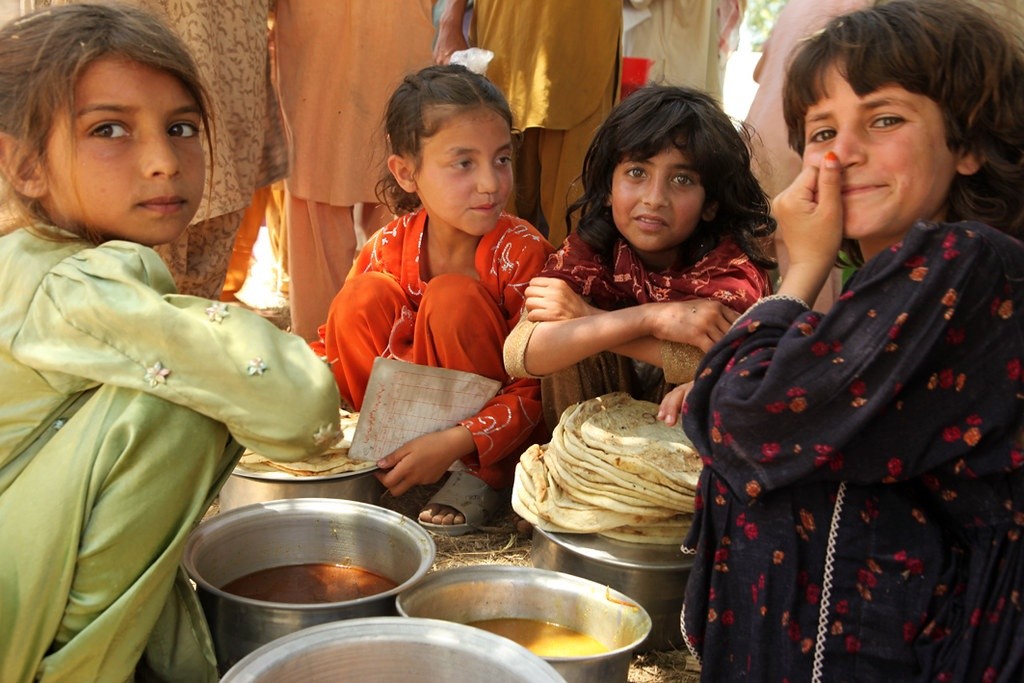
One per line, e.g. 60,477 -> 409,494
621,56 -> 655,102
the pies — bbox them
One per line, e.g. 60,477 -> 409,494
508,393 -> 703,544
238,409 -> 377,475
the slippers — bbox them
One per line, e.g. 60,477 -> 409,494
417,469 -> 503,535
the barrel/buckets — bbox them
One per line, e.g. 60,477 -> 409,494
531,522 -> 696,652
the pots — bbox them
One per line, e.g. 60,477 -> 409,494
180,497 -> 437,678
219,459 -> 385,514
218,615 -> 568,682
397,564 -> 652,683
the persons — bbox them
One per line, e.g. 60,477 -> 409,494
308,66 -> 555,535
0,0 -> 1024,683
